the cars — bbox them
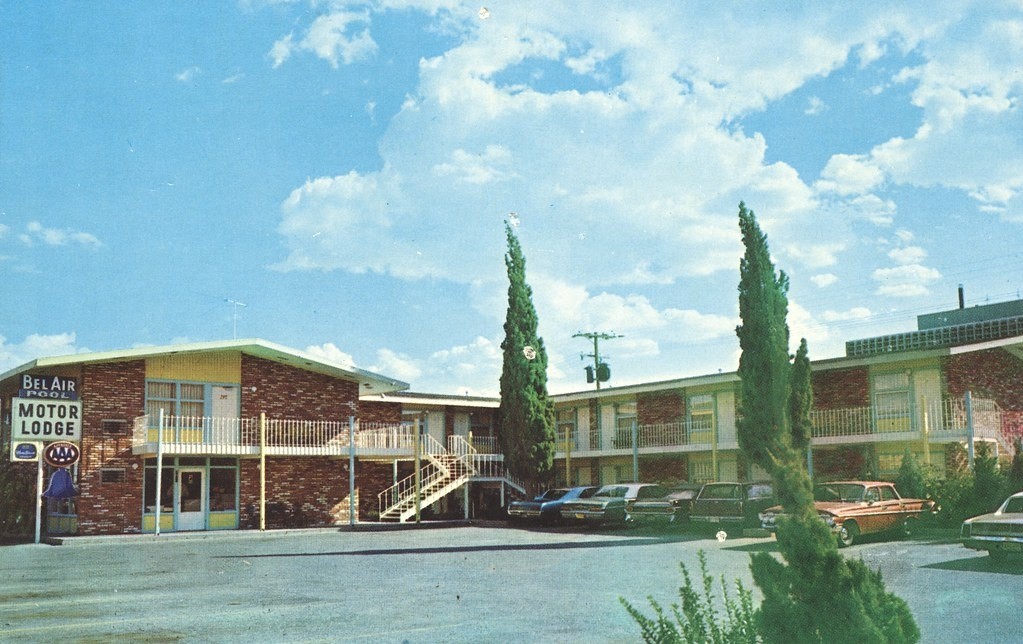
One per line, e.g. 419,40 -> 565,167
624,487 -> 700,530
958,492 -> 1023,560
760,480 -> 939,549
559,482 -> 670,529
506,485 -> 600,524
687,481 -> 778,534
